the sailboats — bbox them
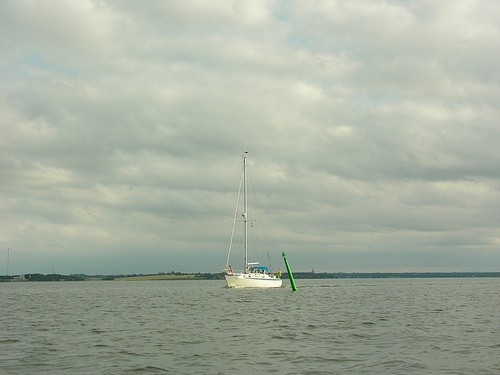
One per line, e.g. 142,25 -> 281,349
222,152 -> 282,289
